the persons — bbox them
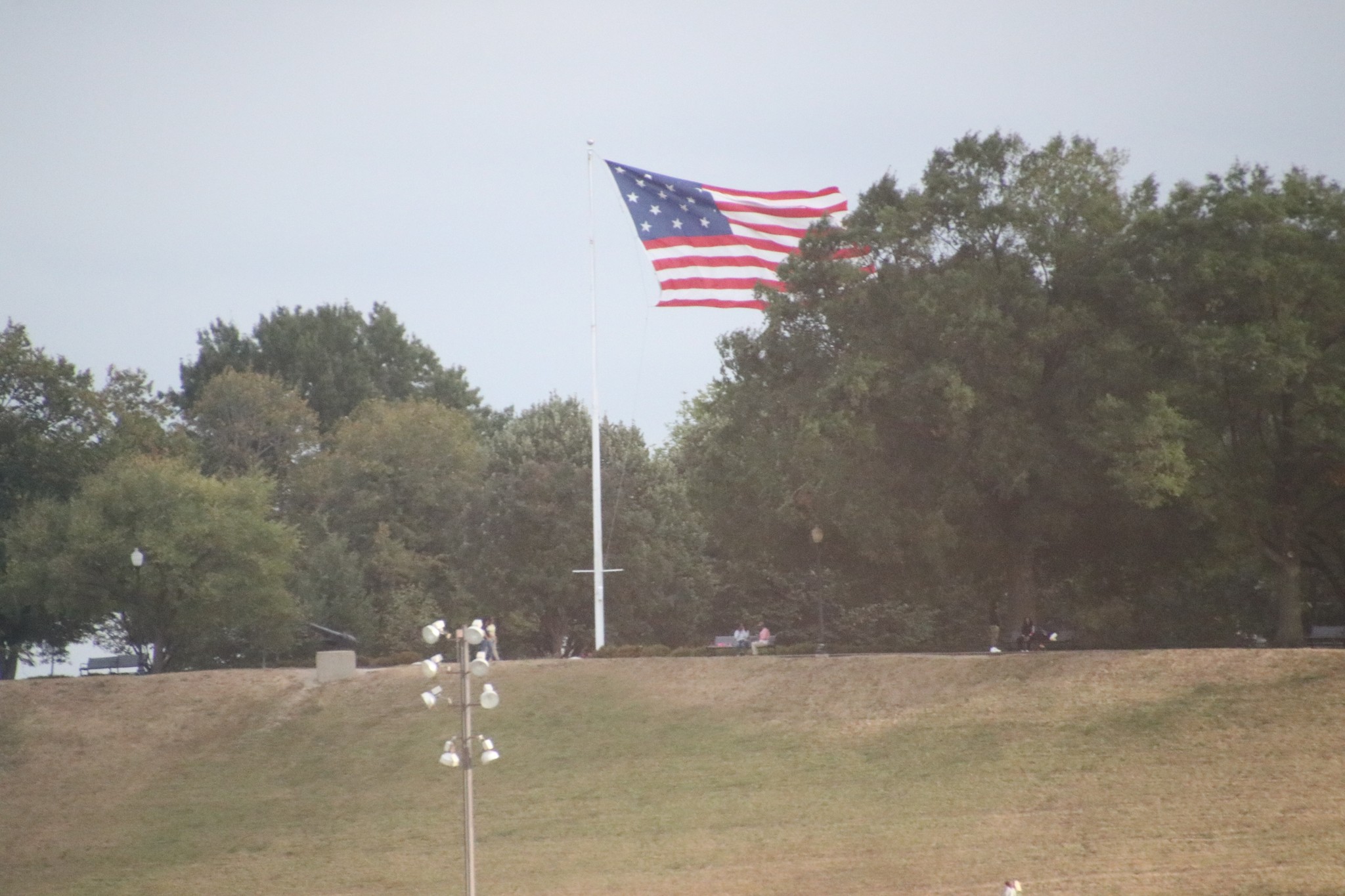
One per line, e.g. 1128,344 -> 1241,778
729,622 -> 751,656
479,618 -> 491,660
750,621 -> 770,656
1016,616 -> 1036,652
486,616 -> 501,660
988,599 -> 1002,652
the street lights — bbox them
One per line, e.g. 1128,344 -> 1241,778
131,547 -> 144,675
811,524 -> 827,653
418,619 -> 501,896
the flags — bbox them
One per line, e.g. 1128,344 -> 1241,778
603,156 -> 878,310
